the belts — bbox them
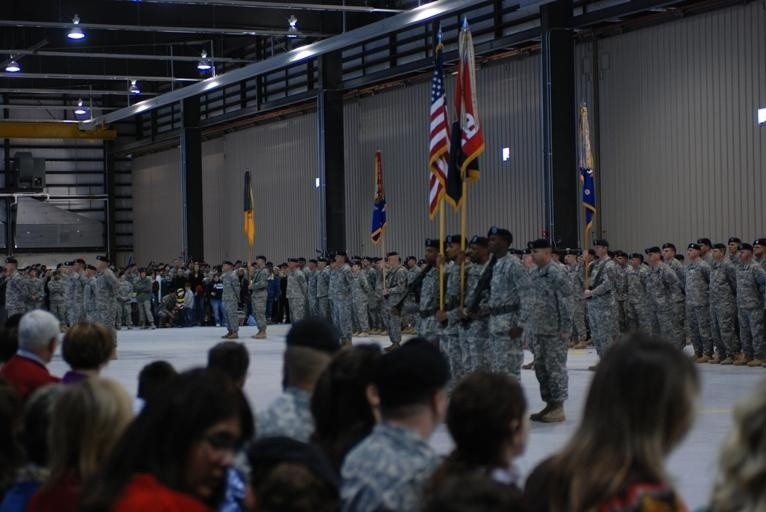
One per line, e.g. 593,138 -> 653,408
488,305 -> 518,315
418,308 -> 438,318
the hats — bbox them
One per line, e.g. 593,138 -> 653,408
286,316 -> 341,353
510,238 -> 643,262
287,251 -> 416,265
425,226 -> 513,248
0,255 -> 109,276
256,255 -> 266,261
374,338 -> 450,408
645,237 -> 766,260
223,260 -> 234,265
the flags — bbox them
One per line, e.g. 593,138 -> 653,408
576,105 -> 598,232
242,169 -> 257,246
446,27 -> 486,211
428,45 -> 450,223
371,147 -> 388,241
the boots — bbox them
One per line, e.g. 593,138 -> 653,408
690,350 -> 766,368
530,400 -> 565,422
221,329 -> 238,338
351,326 -> 417,337
383,342 -> 400,352
252,330 -> 267,339
567,338 -> 593,349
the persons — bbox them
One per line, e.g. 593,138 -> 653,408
1,224 -> 766,510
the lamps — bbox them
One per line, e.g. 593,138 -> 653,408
66,14 -> 85,40
128,79 -> 141,94
5,53 -> 21,72
74,99 -> 86,115
287,14 -> 298,39
197,49 -> 212,70
